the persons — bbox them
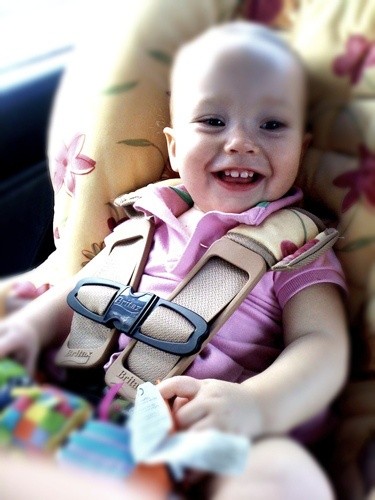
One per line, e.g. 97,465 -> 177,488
0,21 -> 351,500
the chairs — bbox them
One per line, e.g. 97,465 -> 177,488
1,0 -> 375,500
